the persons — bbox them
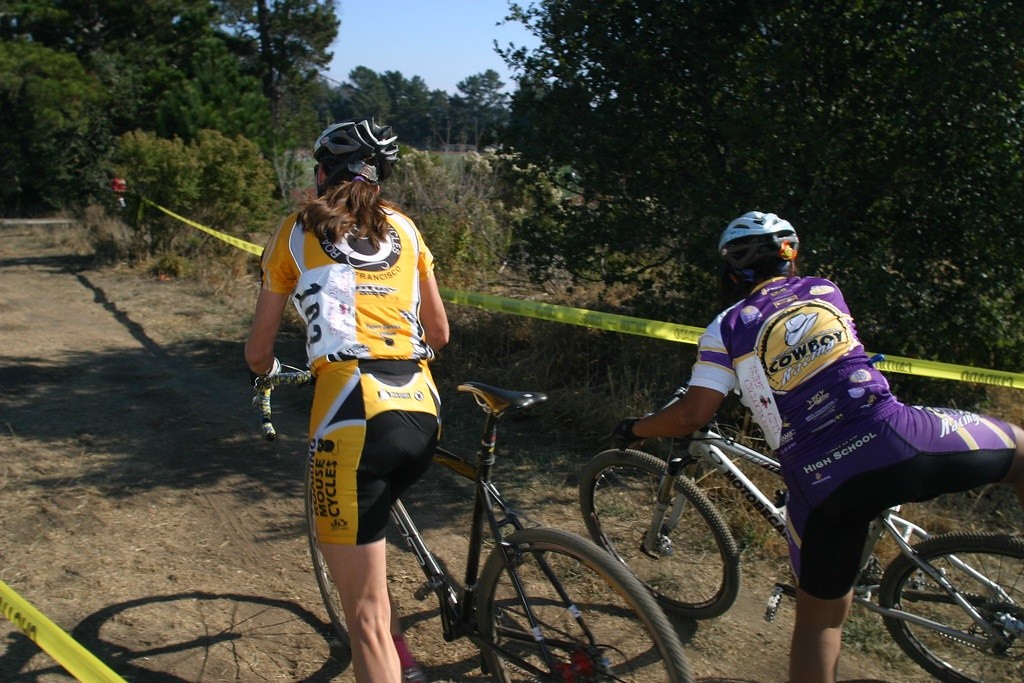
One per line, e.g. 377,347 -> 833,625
612,209 -> 1024,682
245,120 -> 449,683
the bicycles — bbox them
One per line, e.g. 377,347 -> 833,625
579,379 -> 1023,683
251,362 -> 695,683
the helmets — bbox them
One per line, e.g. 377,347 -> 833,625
312,116 -> 399,170
718,210 -> 798,270
